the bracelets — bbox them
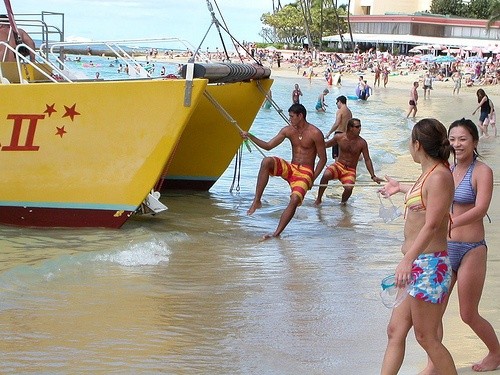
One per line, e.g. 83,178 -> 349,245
371,175 -> 375,178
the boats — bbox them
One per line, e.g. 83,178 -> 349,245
0,0 -> 274,230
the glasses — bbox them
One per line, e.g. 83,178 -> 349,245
350,125 -> 362,128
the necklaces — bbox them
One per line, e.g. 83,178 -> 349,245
297,123 -> 310,140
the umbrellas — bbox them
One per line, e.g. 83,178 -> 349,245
408,43 -> 500,57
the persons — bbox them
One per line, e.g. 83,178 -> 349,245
315,88 -> 329,112
47,43 -> 285,85
0,13 -> 35,64
292,83 -> 303,104
325,95 -> 352,159
314,118 -> 385,207
240,104 -> 327,240
407,45 -> 500,141
417,117 -> 500,375
287,44 -> 408,101
406,81 -> 419,118
376,118 -> 457,375
